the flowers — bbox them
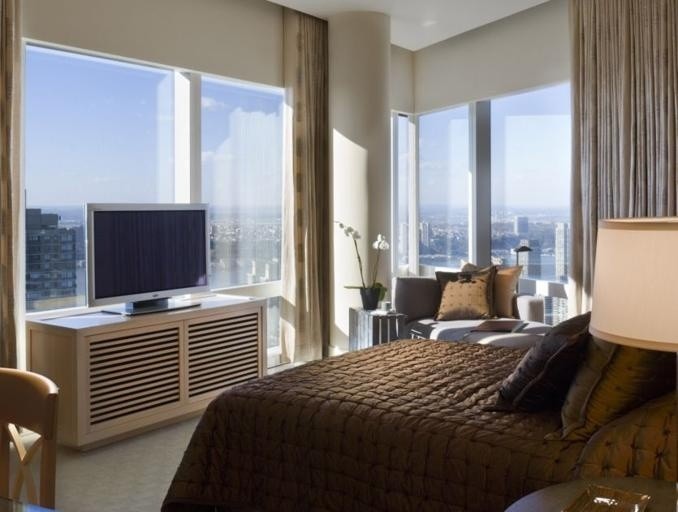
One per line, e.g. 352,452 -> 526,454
335,220 -> 390,288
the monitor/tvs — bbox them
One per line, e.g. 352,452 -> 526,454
83,201 -> 212,317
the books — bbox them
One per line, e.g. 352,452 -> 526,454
470,318 -> 523,333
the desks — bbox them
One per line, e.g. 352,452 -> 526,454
503,474 -> 678,512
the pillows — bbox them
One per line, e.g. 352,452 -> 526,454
459,263 -> 523,318
557,331 -> 633,443
479,312 -> 592,413
436,268 -> 493,320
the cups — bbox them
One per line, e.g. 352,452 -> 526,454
381,301 -> 391,312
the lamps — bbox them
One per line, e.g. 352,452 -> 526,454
592,216 -> 678,352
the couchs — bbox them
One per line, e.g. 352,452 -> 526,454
394,265 -> 560,349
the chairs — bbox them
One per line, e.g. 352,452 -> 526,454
0,367 -> 59,512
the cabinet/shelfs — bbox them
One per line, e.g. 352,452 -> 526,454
24,292 -> 268,450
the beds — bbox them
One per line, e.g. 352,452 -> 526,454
174,338 -> 678,512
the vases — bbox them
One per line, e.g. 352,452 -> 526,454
359,288 -> 380,309
